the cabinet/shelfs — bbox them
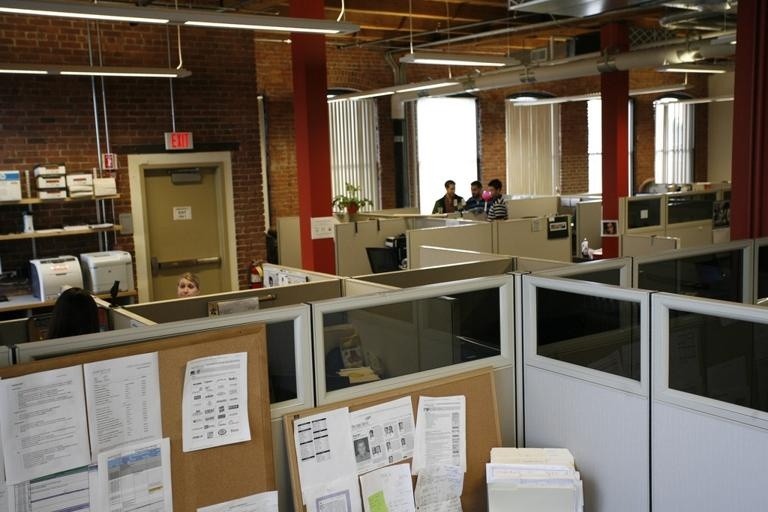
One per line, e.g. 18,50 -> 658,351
0,191 -> 136,317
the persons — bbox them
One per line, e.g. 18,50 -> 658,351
465,181 -> 486,209
432,180 -> 466,213
485,179 -> 507,222
605,222 -> 615,235
173,271 -> 201,299
356,439 -> 369,461
46,286 -> 102,339
268,276 -> 274,287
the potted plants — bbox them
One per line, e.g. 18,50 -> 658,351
330,182 -> 374,214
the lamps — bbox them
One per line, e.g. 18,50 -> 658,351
0,0 -> 180,30
655,4 -> 727,74
399,1 -> 517,68
326,2 -> 459,104
626,73 -> 692,96
183,1 -> 360,37
0,24 -> 192,80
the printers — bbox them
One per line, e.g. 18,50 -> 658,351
29,253 -> 84,303
80,250 -> 135,296
